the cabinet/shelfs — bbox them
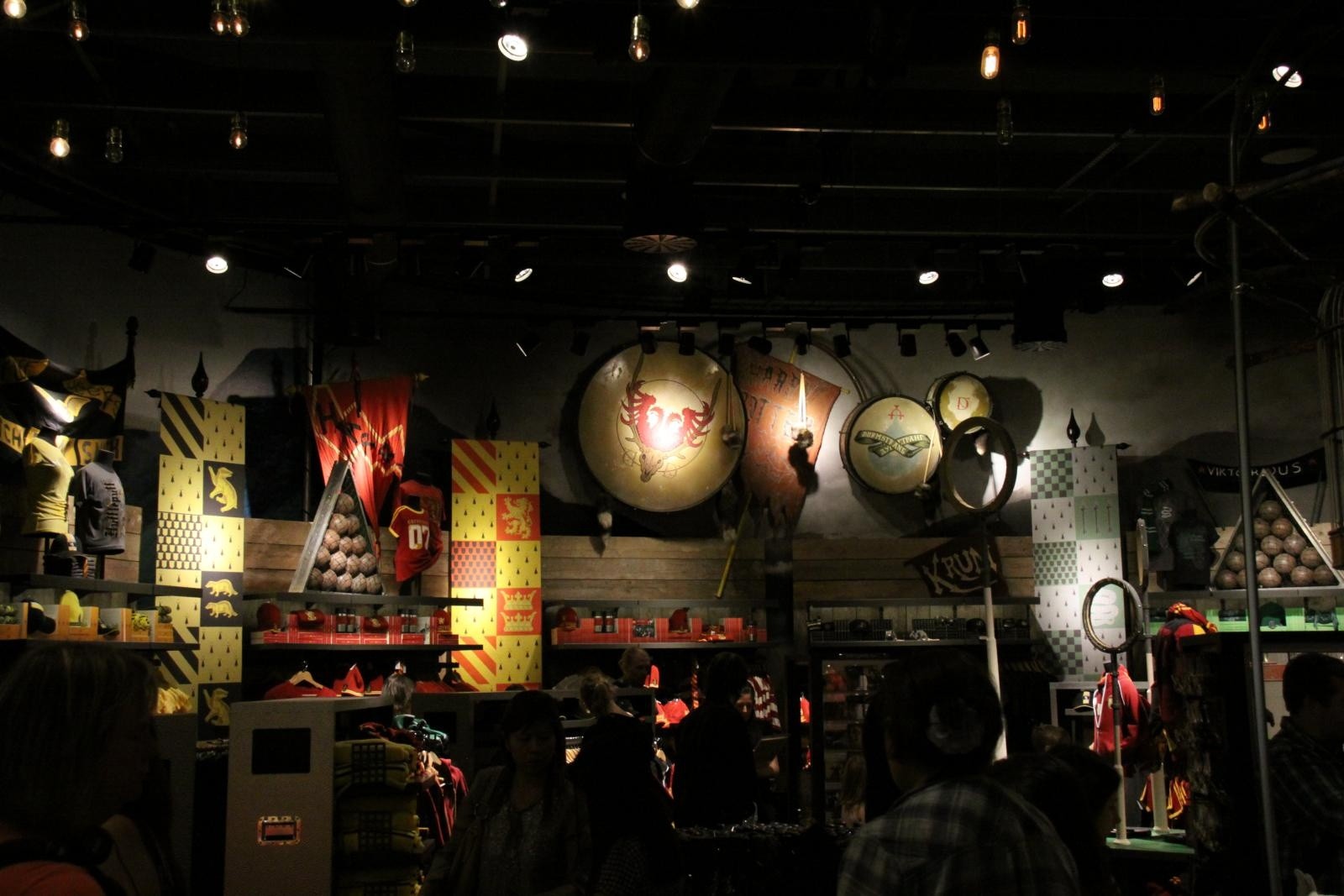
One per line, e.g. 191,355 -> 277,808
2,572 -> 205,652
542,597 -> 770,654
245,587 -> 487,661
222,693 -> 403,894
413,685 -> 663,764
809,588 -> 1042,652
1141,584 -> 1344,643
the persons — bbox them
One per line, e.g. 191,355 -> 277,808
394,470 -> 447,539
1,639 -> 165,894
387,493 -> 445,584
859,586 -> 1119,857
390,642 -> 870,825
834,662 -> 1080,894
419,689 -> 593,895
1164,509 -> 1219,587
1268,655 -> 1344,895
68,448 -> 126,554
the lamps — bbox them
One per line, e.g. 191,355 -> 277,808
4,0 -> 1308,369
